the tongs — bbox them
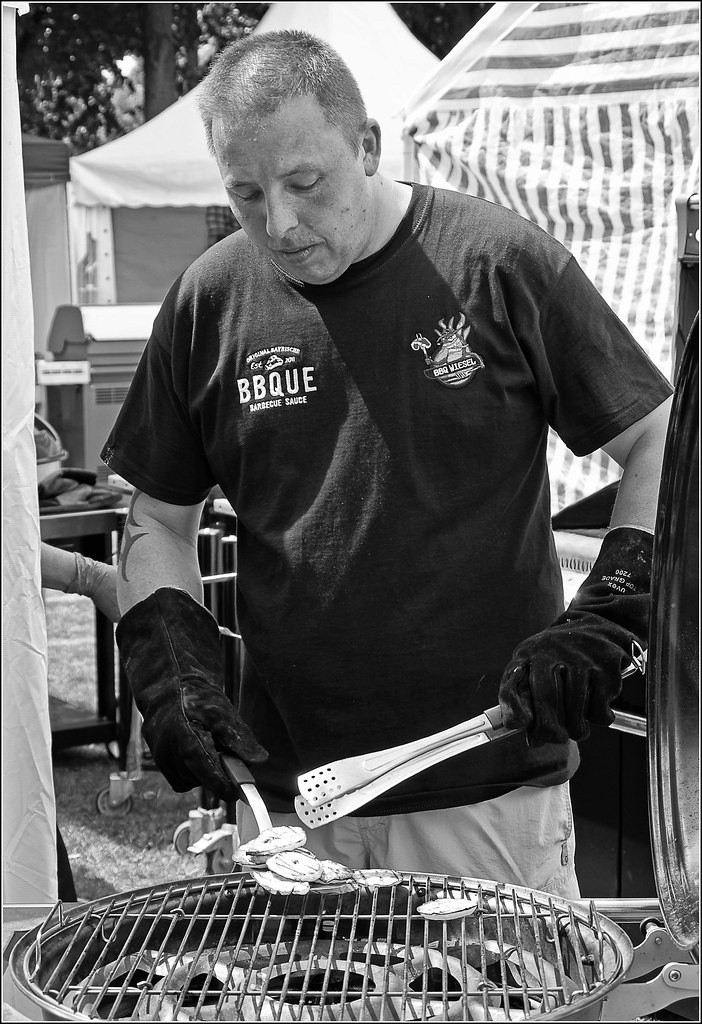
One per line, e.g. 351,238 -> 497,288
287,640 -> 653,832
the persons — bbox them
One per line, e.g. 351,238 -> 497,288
98,30 -> 672,900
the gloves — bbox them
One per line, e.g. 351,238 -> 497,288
115,586 -> 269,804
39,484 -> 122,515
63,551 -> 122,625
38,468 -> 97,498
498,524 -> 655,748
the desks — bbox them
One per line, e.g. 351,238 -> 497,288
41,484 -> 132,770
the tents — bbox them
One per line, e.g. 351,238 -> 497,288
404,1 -> 700,517
69,1 -> 439,300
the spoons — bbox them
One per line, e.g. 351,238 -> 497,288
218,748 -> 311,896
